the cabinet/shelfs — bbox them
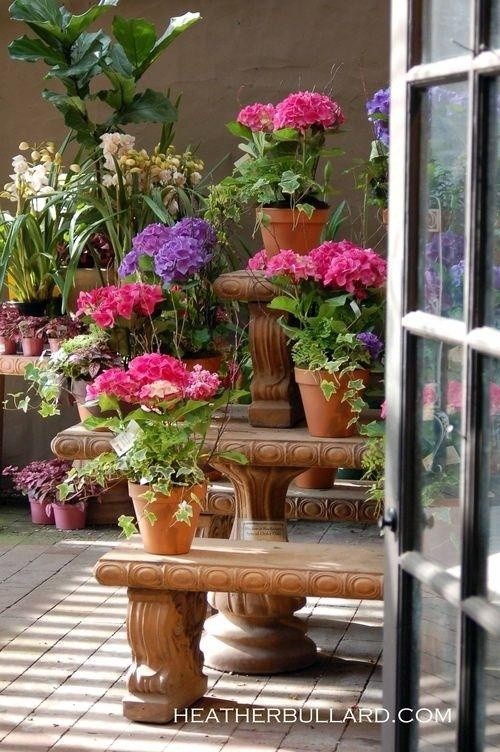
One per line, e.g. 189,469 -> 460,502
91,539 -> 395,731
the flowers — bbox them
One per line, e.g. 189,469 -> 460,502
0,0 -> 500,569
